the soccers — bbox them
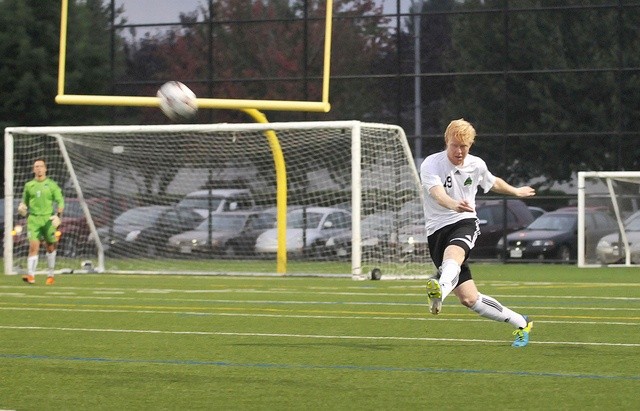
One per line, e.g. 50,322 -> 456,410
155,80 -> 198,118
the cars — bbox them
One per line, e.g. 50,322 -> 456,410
168,213 -> 263,257
596,211 -> 640,263
11,197 -> 110,257
87,204 -> 207,257
497,207 -> 621,263
254,206 -> 353,257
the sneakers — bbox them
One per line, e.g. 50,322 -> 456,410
46,277 -> 55,286
426,279 -> 442,315
22,275 -> 35,284
512,315 -> 533,348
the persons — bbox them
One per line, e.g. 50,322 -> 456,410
420,118 -> 536,348
18,161 -> 65,286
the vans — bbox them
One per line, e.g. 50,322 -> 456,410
179,189 -> 255,211
390,198 -> 534,258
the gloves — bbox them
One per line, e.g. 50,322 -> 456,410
18,202 -> 28,217
50,212 -> 61,227
